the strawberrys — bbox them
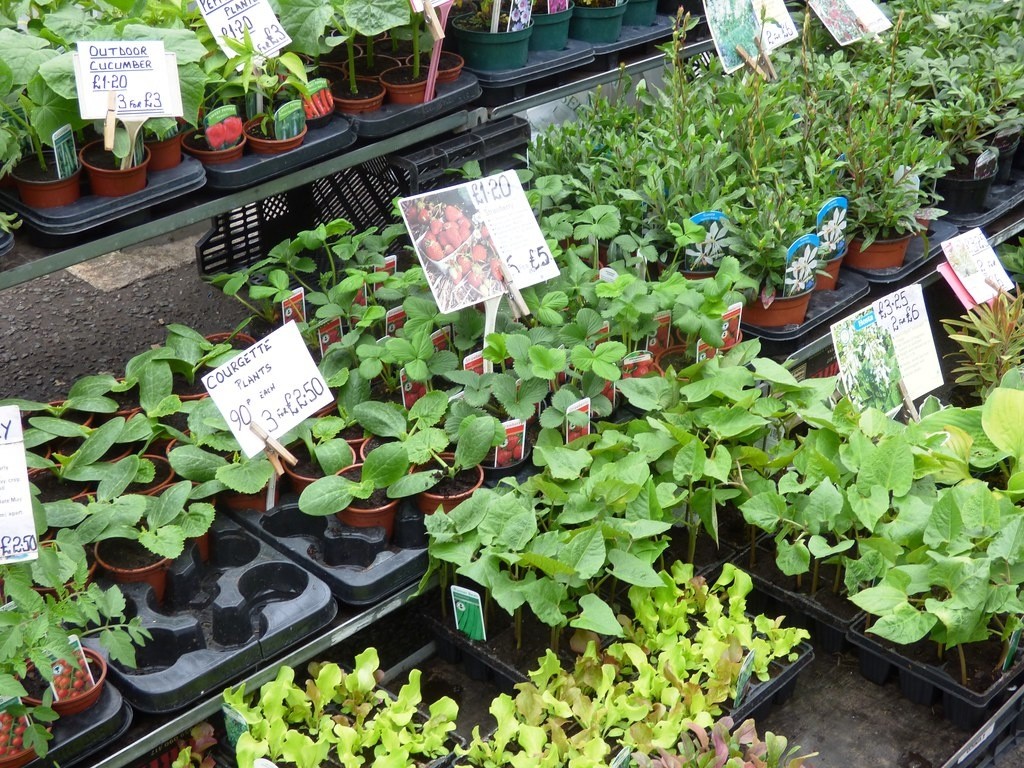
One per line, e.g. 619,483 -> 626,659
407,204 -> 504,286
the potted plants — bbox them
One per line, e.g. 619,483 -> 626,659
0,1 -> 1024,768
